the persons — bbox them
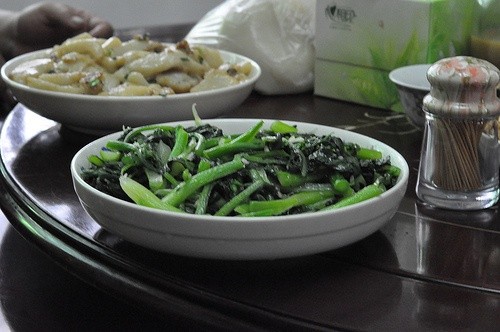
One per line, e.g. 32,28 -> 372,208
0,0 -> 114,119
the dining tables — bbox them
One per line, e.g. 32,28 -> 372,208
0,20 -> 500,332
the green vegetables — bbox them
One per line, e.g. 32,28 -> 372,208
80,103 -> 400,216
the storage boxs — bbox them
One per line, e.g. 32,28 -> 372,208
312,0 -> 500,115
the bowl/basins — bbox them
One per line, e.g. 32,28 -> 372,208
390,62 -> 500,131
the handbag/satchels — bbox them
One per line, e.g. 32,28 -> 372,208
183,0 -> 316,95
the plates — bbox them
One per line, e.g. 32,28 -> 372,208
70,118 -> 411,260
0,46 -> 263,136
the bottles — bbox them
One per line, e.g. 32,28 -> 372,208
416,56 -> 499,211
